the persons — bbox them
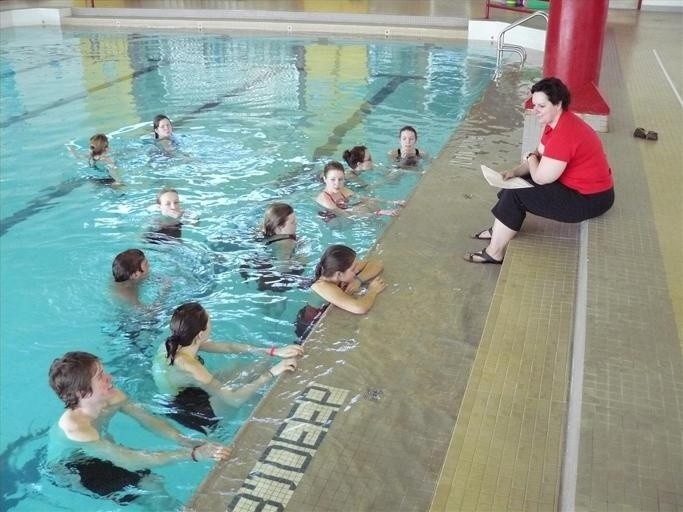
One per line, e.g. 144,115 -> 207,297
139,187 -> 200,245
44,350 -> 233,511
106,248 -> 171,316
141,114 -> 189,171
386,126 -> 439,178
150,302 -> 306,438
64,133 -> 125,198
291,244 -> 386,345
310,146 -> 428,191
238,202 -> 311,343
313,160 -> 407,228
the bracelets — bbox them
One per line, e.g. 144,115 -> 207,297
353,275 -> 363,284
266,369 -> 275,378
270,347 -> 275,356
190,445 -> 200,462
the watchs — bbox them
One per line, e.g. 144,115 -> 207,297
526,153 -> 539,163
375,208 -> 381,216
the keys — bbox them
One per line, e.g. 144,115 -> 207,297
461,76 -> 615,263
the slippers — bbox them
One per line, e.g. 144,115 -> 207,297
463,249 -> 504,264
471,227 -> 493,239
